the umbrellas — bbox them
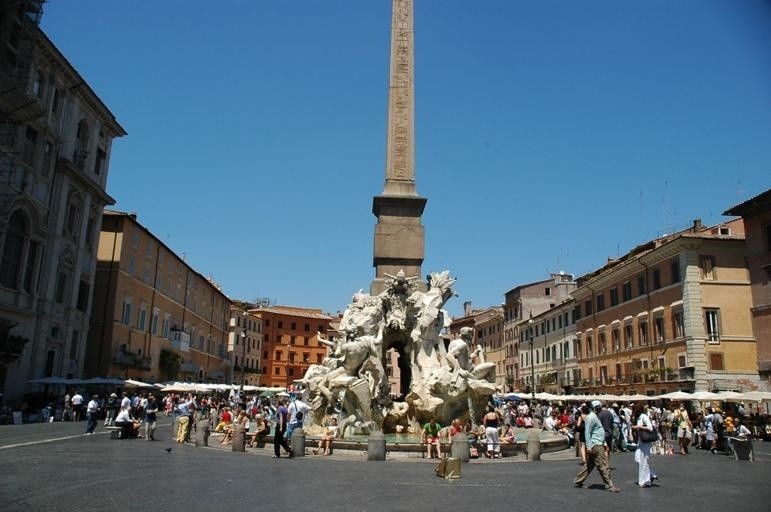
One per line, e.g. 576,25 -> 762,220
493,390 -> 771,415
24,375 -> 286,399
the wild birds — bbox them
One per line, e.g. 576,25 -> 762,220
165,447 -> 172,453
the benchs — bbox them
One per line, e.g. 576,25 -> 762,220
106,427 -> 123,440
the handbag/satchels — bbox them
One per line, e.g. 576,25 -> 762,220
640,429 -> 657,443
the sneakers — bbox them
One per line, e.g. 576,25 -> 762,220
606,486 -> 620,493
572,485 -> 588,489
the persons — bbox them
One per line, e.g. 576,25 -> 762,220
293,331 -> 339,384
317,321 -> 386,408
630,404 -> 658,487
1,389 -> 342,459
573,400 -> 620,493
445,326 -> 502,389
418,401 -> 771,463
